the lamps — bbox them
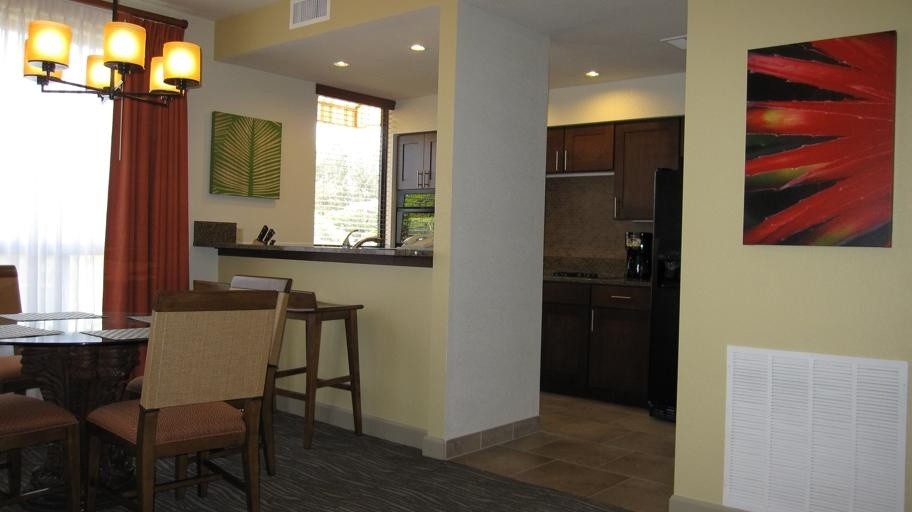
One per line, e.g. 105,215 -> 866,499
24,1 -> 202,159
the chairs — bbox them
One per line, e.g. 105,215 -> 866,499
0,393 -> 79,512
126,276 -> 291,499
274,291 -> 363,450
85,289 -> 278,512
0,265 -> 38,396
588,282 -> 650,411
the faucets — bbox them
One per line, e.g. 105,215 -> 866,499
353,236 -> 382,248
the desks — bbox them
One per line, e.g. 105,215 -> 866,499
0,311 -> 153,507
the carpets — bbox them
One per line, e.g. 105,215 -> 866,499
0,410 -> 629,511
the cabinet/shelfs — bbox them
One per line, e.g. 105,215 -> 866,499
396,131 -> 437,190
540,279 -> 591,400
611,114 -> 681,221
545,120 -> 616,175
